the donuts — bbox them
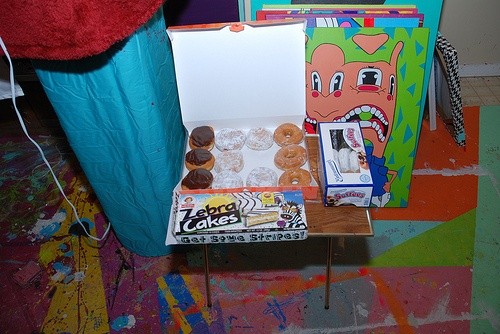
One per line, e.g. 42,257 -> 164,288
273,123 -> 303,146
278,168 -> 311,186
274,144 -> 308,170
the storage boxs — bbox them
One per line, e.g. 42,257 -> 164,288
166,17 -> 375,244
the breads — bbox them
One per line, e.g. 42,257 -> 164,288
245,129 -> 273,150
181,125 -> 215,191
214,150 -> 245,173
215,128 -> 246,151
245,167 -> 278,187
213,170 -> 243,188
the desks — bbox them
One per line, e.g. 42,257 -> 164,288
165,137 -> 374,310
